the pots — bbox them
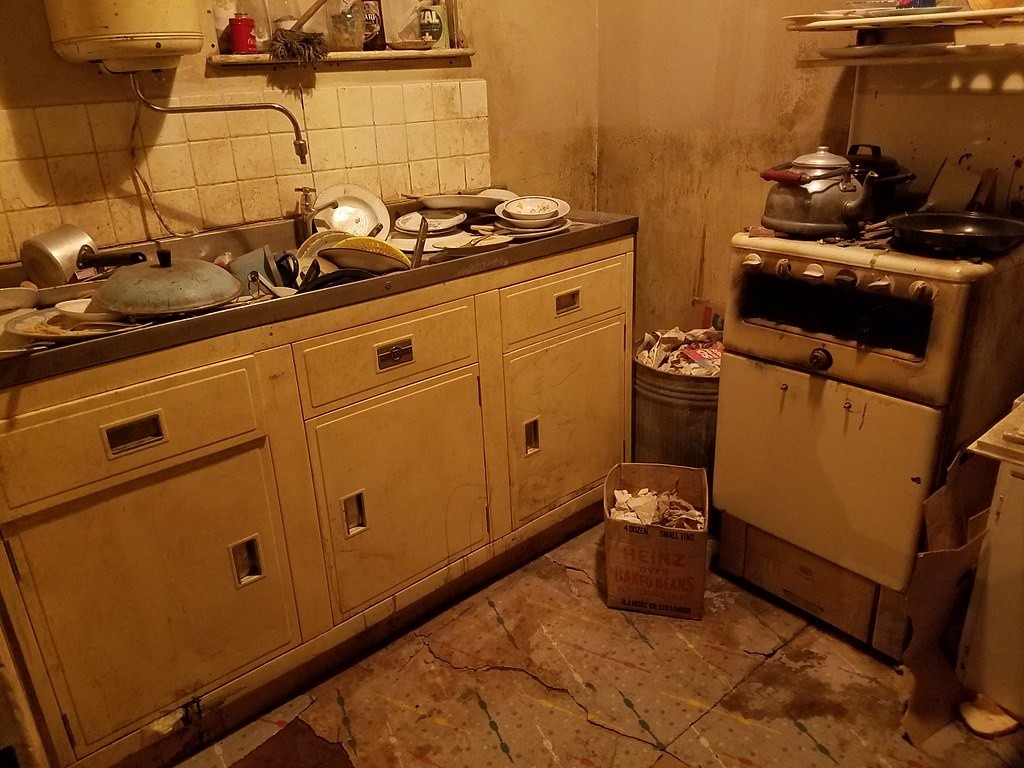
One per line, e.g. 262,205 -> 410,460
22,223 -> 148,286
889,211 -> 1024,257
843,144 -> 916,219
866,152 -> 982,232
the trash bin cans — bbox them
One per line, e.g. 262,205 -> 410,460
633,331 -> 720,473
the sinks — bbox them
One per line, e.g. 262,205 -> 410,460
0,183 -> 603,391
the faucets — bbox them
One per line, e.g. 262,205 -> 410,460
295,187 -> 339,239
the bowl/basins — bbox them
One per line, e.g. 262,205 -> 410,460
505,198 -> 558,219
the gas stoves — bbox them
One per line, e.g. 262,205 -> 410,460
713,222 -> 994,588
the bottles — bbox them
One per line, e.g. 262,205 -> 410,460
230,11 -> 257,54
362,0 -> 386,50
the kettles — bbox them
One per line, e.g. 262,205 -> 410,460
760,147 -> 879,236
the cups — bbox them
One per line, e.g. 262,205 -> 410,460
324,0 -> 365,52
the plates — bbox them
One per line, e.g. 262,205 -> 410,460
292,230 -> 412,272
479,189 -> 521,198
316,183 -> 390,242
56,300 -> 126,318
39,279 -> 107,302
782,8 -> 1024,56
497,195 -> 570,226
394,210 -> 467,236
387,40 -> 438,49
9,308 -> 150,336
493,219 -> 570,240
0,289 -> 40,310
432,232 -> 515,256
418,195 -> 502,208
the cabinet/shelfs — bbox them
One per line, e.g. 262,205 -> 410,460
786,7 -> 1024,68
0,216 -> 639,768
953,460 -> 1024,720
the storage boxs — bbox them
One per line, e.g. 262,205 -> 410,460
603,462 -> 708,621
